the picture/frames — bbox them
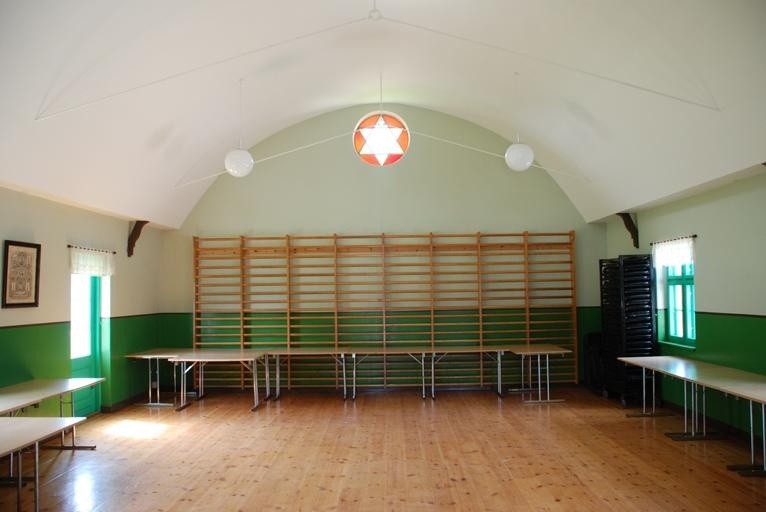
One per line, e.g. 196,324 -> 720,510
2,240 -> 41,308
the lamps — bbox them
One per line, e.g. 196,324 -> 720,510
505,71 -> 534,171
223,78 -> 254,177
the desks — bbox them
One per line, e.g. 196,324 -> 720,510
0,378 -> 106,512
617,356 -> 766,476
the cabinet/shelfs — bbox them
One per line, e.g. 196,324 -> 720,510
599,255 -> 663,408
